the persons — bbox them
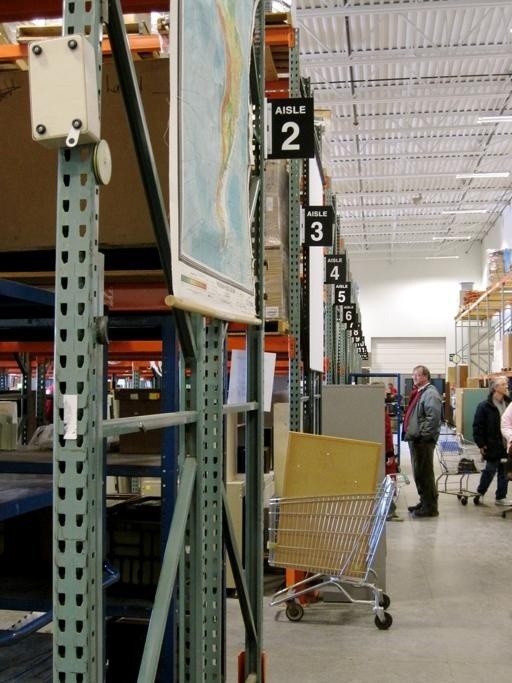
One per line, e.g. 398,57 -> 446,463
385,404 -> 404,522
402,365 -> 441,517
385,383 -> 417,406
473,375 -> 512,507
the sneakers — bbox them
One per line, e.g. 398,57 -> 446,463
495,498 -> 512,506
407,503 -> 439,517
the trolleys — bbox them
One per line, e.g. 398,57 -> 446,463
436,433 -> 486,505
267,474 -> 410,630
